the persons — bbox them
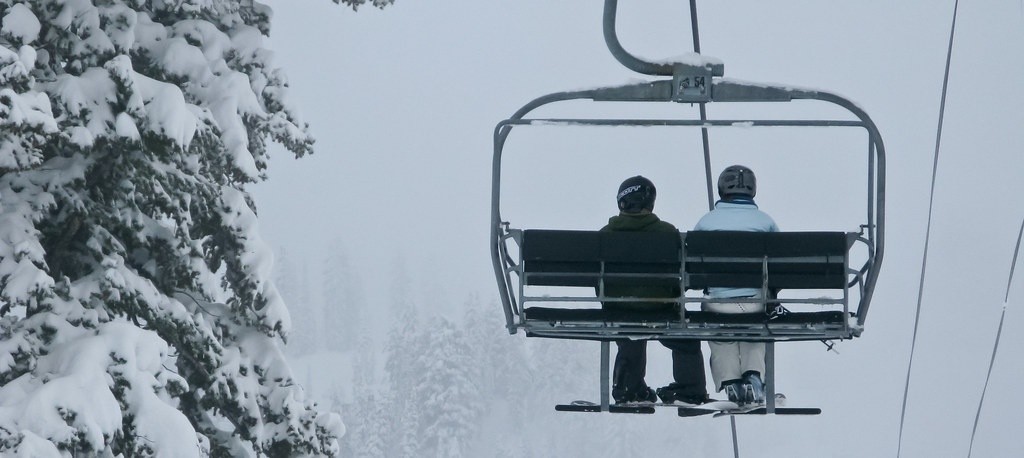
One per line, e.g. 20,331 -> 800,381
693,165 -> 782,404
595,175 -> 708,402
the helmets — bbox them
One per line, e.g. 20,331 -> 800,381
617,175 -> 657,214
717,165 -> 757,199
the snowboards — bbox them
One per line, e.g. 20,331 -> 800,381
555,392 -> 827,418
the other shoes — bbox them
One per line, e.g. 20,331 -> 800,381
656,381 -> 710,405
725,374 -> 766,407
611,378 -> 657,404
